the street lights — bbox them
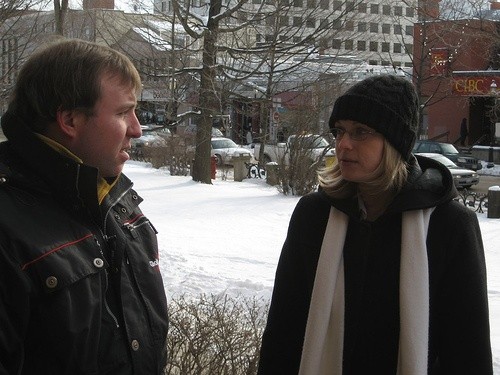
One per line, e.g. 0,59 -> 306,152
139,84 -> 144,122
487,78 -> 498,169
168,77 -> 177,140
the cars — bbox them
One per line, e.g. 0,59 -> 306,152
412,141 -> 482,190
131,124 -> 252,166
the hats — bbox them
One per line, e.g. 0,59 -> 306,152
329,75 -> 420,157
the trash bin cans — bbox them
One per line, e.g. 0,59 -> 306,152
211,154 -> 217,179
232,151 -> 252,182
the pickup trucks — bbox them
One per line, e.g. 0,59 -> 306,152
254,134 -> 336,169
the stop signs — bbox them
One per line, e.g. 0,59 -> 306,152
274,112 -> 279,121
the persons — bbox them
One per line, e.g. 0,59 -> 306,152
258,75 -> 493,375
0,39 -> 169,375
460,118 -> 467,147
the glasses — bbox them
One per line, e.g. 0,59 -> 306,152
330,126 -> 378,139
